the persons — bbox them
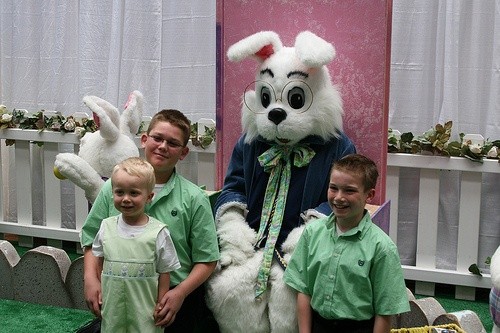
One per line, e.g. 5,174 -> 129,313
79,109 -> 220,333
284,155 -> 410,333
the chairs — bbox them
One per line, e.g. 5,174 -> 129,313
197,0 -> 394,333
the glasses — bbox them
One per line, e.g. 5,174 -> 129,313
148,135 -> 184,148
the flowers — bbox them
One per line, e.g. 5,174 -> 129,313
388,120 -> 500,165
0,104 -> 216,151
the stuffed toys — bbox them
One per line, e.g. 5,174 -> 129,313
488,246 -> 500,333
53,91 -> 142,209
206,30 -> 356,333
